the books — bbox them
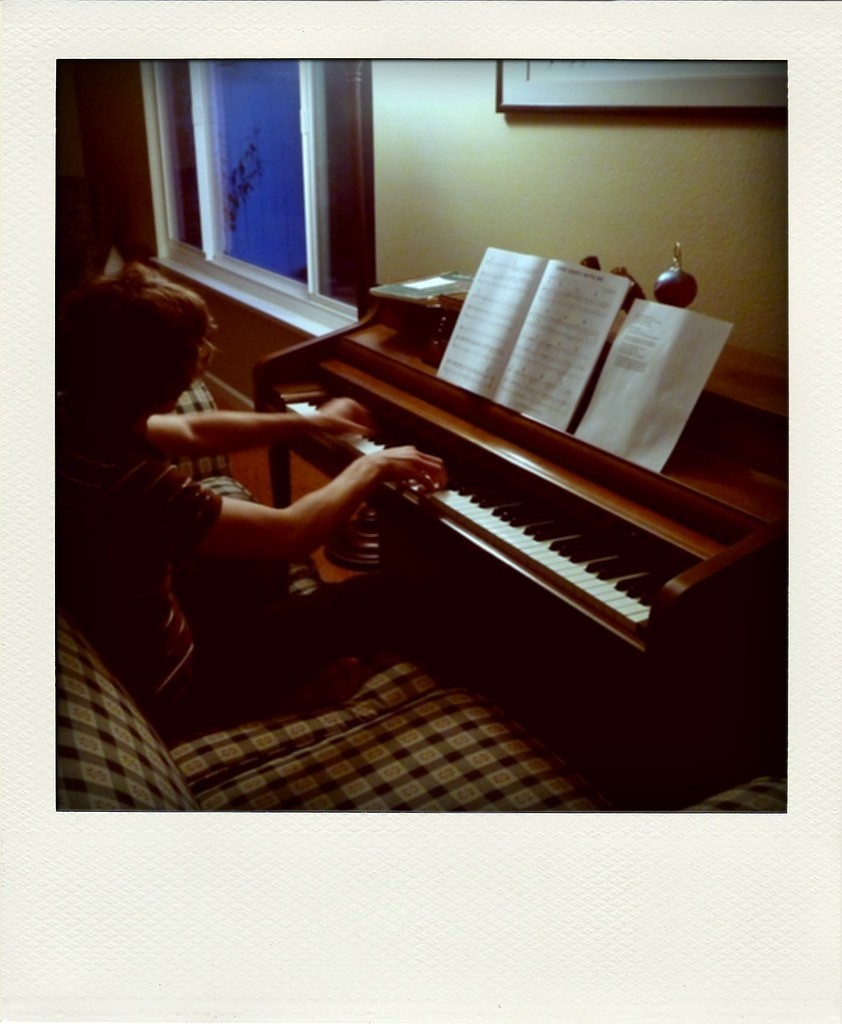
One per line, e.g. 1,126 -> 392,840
434,245 -> 634,432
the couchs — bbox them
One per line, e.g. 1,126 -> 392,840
55,380 -> 787,812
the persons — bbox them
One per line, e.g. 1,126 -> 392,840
55,263 -> 443,726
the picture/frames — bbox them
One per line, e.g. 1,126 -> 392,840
497,60 -> 787,118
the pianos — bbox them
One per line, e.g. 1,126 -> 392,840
250,271 -> 789,659
